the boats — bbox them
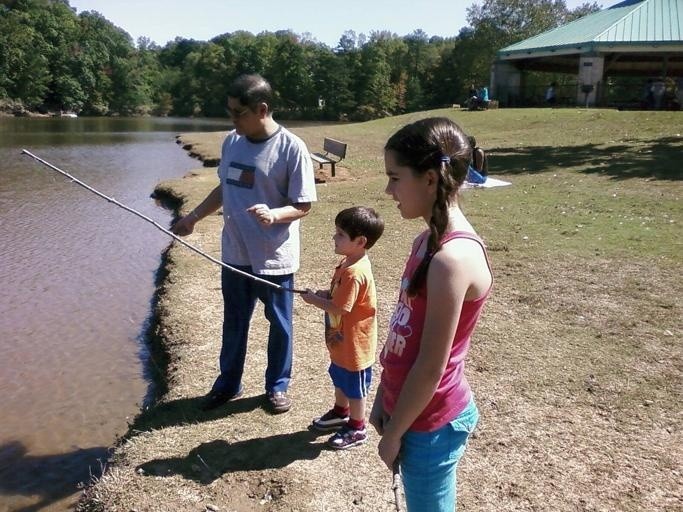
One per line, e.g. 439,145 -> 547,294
50,113 -> 78,120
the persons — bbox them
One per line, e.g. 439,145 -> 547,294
466,135 -> 487,183
479,86 -> 491,110
546,82 -> 557,106
467,84 -> 479,110
172,74 -> 317,414
369,117 -> 494,512
301,206 -> 385,450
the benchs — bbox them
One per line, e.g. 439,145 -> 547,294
308,135 -> 348,178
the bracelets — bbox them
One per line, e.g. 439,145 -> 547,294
191,209 -> 200,222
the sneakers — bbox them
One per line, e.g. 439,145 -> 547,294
265,390 -> 292,414
313,409 -> 350,433
197,385 -> 244,412
326,422 -> 368,449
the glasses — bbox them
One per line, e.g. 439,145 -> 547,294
225,102 -> 257,117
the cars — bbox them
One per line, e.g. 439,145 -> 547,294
638,75 -> 678,102
